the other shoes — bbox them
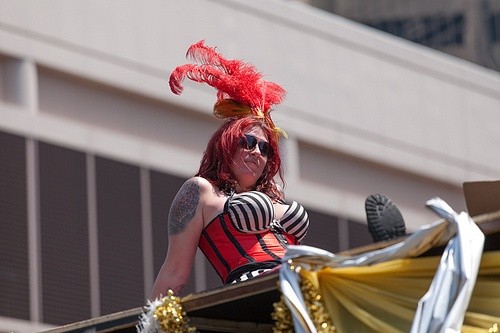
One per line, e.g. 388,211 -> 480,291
364,192 -> 406,244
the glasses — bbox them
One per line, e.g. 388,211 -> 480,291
237,134 -> 275,158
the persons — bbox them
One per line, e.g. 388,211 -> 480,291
151,113 -> 406,306
